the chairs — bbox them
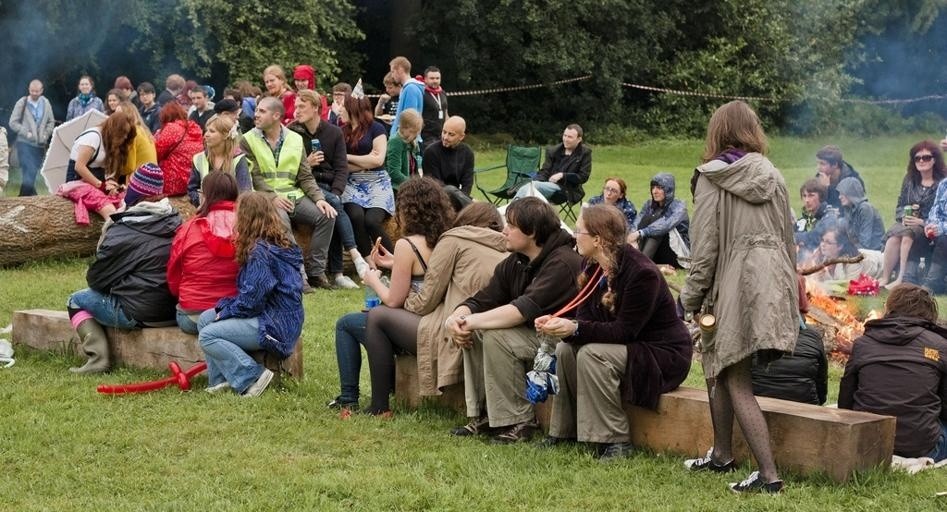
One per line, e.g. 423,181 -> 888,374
472,147 -> 543,201
550,191 -> 585,223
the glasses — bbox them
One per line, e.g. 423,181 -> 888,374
913,155 -> 935,162
572,232 -> 592,239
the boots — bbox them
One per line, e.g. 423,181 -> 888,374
69,318 -> 112,376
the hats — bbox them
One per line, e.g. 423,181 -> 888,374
124,162 -> 165,206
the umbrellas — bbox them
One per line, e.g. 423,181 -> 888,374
524,332 -> 561,404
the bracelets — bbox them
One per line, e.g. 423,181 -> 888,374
639,230 -> 641,240
444,315 -> 464,331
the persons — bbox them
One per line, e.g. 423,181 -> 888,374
360,201 -> 513,419
625,175 -> 691,269
196,188 -> 305,396
166,171 -> 241,335
445,196 -> 585,444
1,56 -> 474,292
65,163 -> 186,373
534,204 -> 693,461
680,100 -> 800,492
326,178 -> 457,416
838,285 -> 946,463
586,177 -> 636,231
792,138 -> 946,291
750,310 -> 827,405
494,124 -> 591,216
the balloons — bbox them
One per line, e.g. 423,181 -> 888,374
97,362 -> 208,399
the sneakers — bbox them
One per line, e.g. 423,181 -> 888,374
683,447 -> 737,476
493,416 -> 543,447
309,275 -> 333,290
352,254 -> 371,281
598,443 -> 634,462
449,413 -> 489,438
363,407 -> 393,420
240,367 -> 275,398
325,400 -> 361,413
204,380 -> 229,395
301,280 -> 314,294
545,435 -> 568,446
333,276 -> 360,291
729,470 -> 786,498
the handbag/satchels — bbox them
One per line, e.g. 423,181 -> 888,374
8,147 -> 20,169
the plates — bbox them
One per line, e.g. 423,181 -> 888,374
375,115 -> 396,121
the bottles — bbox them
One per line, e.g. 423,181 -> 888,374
918,256 -> 927,285
699,314 -> 716,332
904,205 -> 912,216
365,285 -> 379,311
912,203 -> 921,218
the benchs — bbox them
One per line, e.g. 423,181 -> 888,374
395,355 -> 896,485
12,310 -> 303,384
